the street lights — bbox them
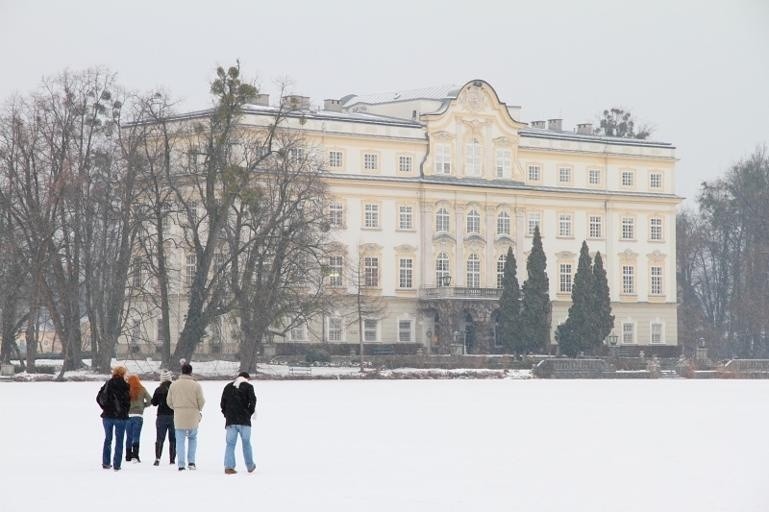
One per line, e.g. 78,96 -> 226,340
322,270 -> 339,341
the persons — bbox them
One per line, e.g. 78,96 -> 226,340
96,365 -> 205,470
221,372 -> 256,474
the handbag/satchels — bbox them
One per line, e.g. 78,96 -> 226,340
99,389 -> 108,406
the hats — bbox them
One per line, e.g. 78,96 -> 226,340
160,369 -> 172,382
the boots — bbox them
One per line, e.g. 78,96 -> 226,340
126,443 -> 141,463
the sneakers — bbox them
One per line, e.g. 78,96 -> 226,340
248,464 -> 256,473
103,465 -> 121,470
153,461 -> 159,466
179,463 -> 196,471
225,469 -> 237,474
170,461 -> 175,464
